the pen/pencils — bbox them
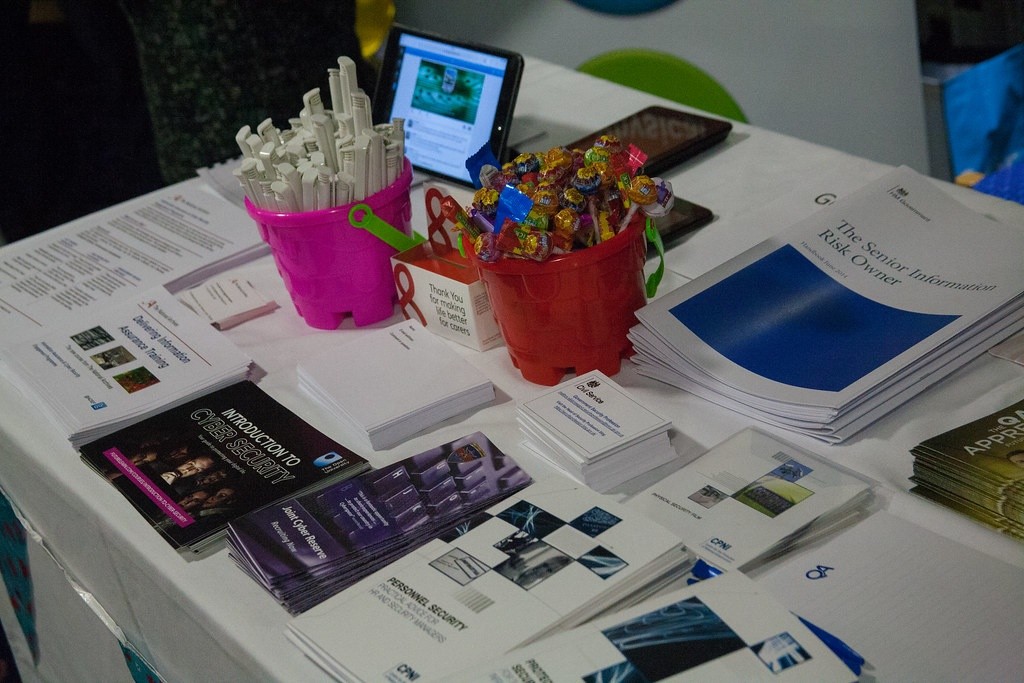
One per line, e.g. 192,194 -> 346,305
232,55 -> 406,211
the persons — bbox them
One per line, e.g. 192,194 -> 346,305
103,433 -> 239,530
1007,450 -> 1024,467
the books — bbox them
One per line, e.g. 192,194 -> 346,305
626,164 -> 1024,445
226,432 -> 865,683
907,398 -> 1024,542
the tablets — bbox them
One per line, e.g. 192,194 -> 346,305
371,22 -> 524,195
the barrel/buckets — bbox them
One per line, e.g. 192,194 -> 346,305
457,208 -> 665,386
243,154 -> 426,331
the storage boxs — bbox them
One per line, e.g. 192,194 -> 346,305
388,180 -> 506,353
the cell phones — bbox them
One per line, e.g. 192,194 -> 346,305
569,105 -> 733,176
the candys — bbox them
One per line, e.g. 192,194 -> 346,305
440,134 -> 675,263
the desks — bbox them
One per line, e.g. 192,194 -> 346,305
0,58 -> 1024,683
